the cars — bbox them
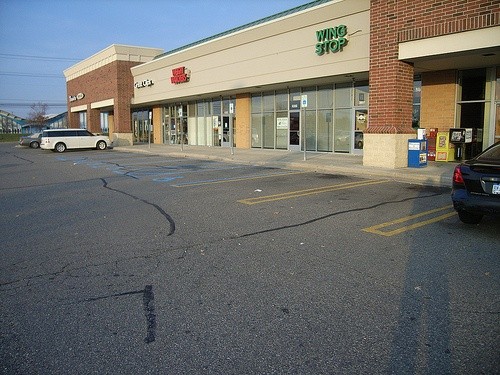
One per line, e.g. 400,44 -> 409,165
451,141 -> 500,223
19,133 -> 41,149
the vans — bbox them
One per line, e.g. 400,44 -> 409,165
40,128 -> 113,153
